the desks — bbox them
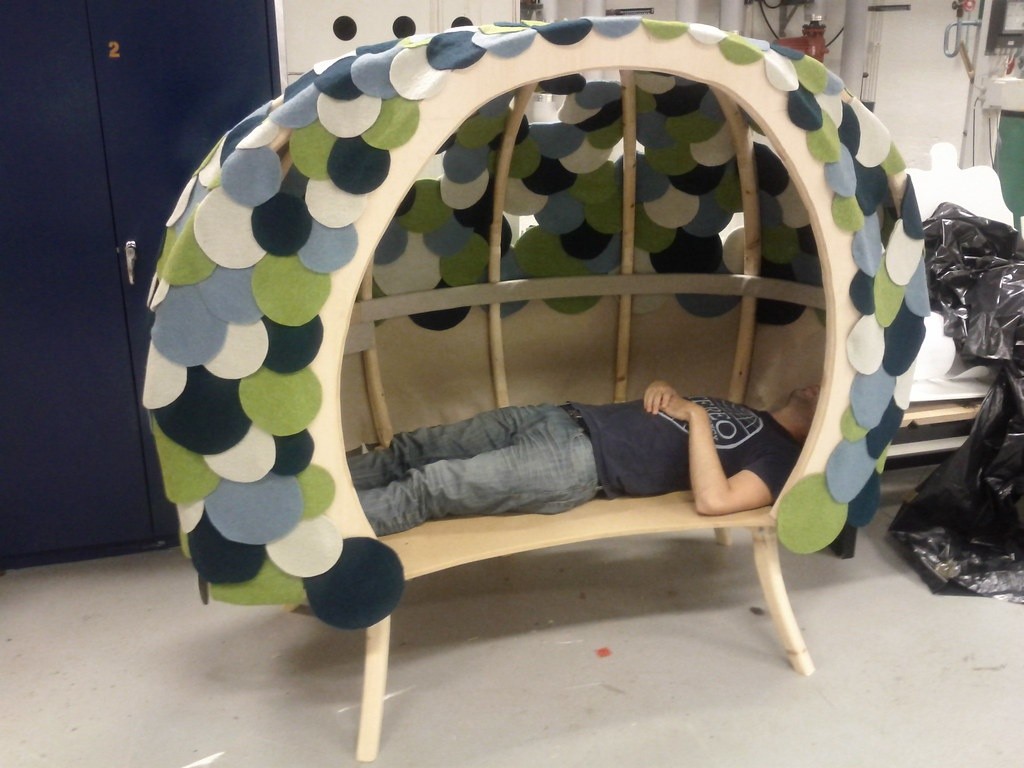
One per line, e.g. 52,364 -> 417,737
829,398 -> 988,559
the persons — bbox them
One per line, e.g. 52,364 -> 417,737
346,381 -> 822,537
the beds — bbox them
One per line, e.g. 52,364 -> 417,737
144,16 -> 934,763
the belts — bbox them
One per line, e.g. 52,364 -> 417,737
562,402 -> 591,440
